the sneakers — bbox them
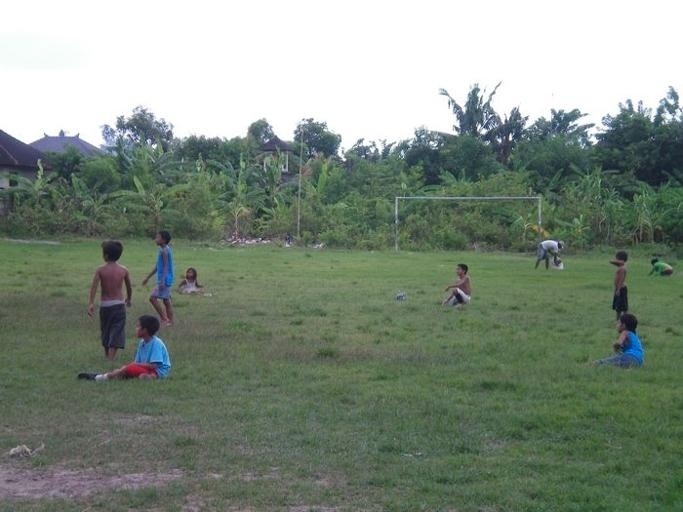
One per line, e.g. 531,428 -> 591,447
78,373 -> 97,381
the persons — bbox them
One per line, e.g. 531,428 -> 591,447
177,266 -> 205,297
608,249 -> 628,321
588,313 -> 644,369
86,239 -> 134,363
125,263 -> 127,266
534,239 -> 566,270
77,316 -> 171,381
139,231 -> 174,332
647,257 -> 674,277
442,264 -> 473,307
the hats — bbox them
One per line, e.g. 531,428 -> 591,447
559,240 -> 564,249
